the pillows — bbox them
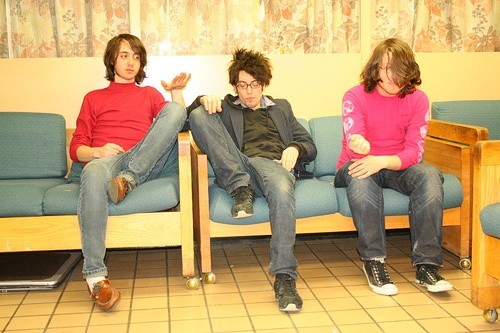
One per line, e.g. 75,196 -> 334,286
0,112 -> 68,179
308,116 -> 343,177
289,118 -> 315,173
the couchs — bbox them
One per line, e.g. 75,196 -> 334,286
432,100 -> 500,316
188,115 -> 471,284
0,112 -> 200,290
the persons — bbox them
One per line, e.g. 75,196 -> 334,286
179,47 -> 318,313
335,38 -> 454,296
69,34 -> 191,313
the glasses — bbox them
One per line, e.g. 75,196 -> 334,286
237,80 -> 260,90
379,65 -> 391,72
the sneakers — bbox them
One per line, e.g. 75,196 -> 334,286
415,264 -> 452,292
231,184 -> 255,218
362,259 -> 398,296
274,272 -> 302,311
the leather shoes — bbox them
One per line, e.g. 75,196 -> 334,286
107,178 -> 126,203
87,276 -> 121,312
